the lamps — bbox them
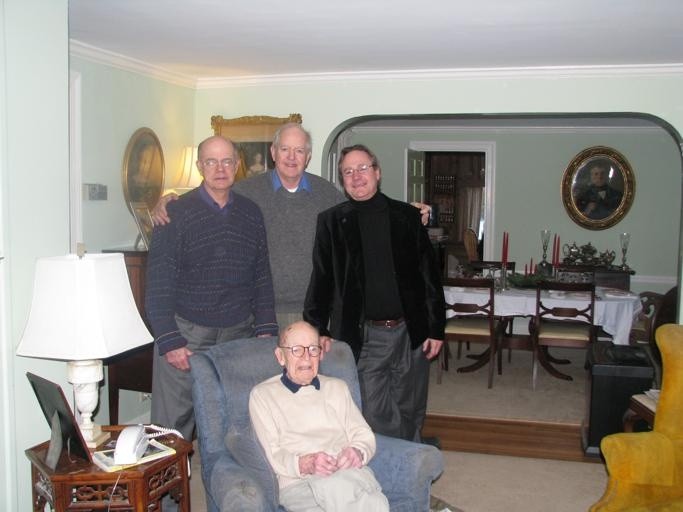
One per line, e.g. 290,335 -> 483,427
540,229 -> 551,266
619,231 -> 633,268
168,143 -> 204,194
15,253 -> 156,449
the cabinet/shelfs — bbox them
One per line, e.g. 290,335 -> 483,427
547,263 -> 636,292
432,175 -> 457,228
101,248 -> 153,440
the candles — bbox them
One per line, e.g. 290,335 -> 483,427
553,233 -> 561,263
500,231 -> 508,266
523,262 -> 529,279
534,263 -> 538,274
529,256 -> 534,275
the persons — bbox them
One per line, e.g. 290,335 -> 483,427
150,136 -> 279,512
245,153 -> 271,177
574,163 -> 622,221
129,144 -> 154,199
303,144 -> 445,442
150,123 -> 434,338
247,321 -> 390,512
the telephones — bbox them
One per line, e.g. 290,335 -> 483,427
112,424 -> 151,466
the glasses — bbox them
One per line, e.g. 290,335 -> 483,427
281,344 -> 323,358
341,165 -> 372,176
200,158 -> 233,167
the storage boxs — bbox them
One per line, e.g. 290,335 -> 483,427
581,342 -> 654,458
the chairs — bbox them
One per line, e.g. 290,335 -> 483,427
462,226 -> 480,263
457,259 -> 516,364
591,291 -> 665,363
532,277 -> 597,382
440,277 -> 509,390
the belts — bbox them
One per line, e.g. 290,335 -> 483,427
368,317 -> 404,328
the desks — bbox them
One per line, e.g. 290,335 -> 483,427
439,242 -> 469,278
621,393 -> 657,433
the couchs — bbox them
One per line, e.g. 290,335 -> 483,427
590,322 -> 683,512
189,334 -> 447,512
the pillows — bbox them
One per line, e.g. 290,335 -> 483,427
224,421 -> 281,511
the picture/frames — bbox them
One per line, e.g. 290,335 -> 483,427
127,199 -> 156,252
561,146 -> 637,232
122,126 -> 168,220
25,370 -> 94,474
210,113 -> 303,182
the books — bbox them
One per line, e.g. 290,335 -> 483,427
644,389 -> 660,403
91,438 -> 177,473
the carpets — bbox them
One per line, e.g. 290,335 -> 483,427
430,449 -> 609,510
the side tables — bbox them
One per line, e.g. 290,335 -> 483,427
25,427 -> 194,512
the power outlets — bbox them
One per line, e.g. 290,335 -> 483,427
138,392 -> 150,405
85,183 -> 108,200
